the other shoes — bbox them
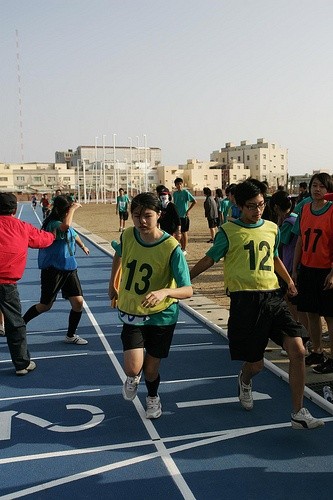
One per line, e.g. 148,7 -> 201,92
181,248 -> 187,256
207,239 -> 214,243
119,228 -> 125,232
281,341 -> 314,355
0,329 -> 6,337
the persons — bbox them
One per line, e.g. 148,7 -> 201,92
243,173 -> 333,374
116,188 -> 131,232
203,183 -> 237,243
156,177 -> 197,256
0,192 -> 56,376
23,195 -> 90,345
32,190 -> 76,221
108,192 -> 194,418
0,311 -> 6,336
190,181 -> 325,432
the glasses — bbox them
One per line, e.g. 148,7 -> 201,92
243,201 -> 267,210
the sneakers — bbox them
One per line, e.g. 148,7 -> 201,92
16,361 -> 36,374
64,334 -> 88,344
123,369 -> 143,401
145,394 -> 161,419
312,358 -> 333,374
304,352 -> 327,366
238,370 -> 254,411
290,408 -> 324,430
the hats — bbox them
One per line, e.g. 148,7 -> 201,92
159,188 -> 170,194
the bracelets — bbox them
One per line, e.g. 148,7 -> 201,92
82,244 -> 85,249
